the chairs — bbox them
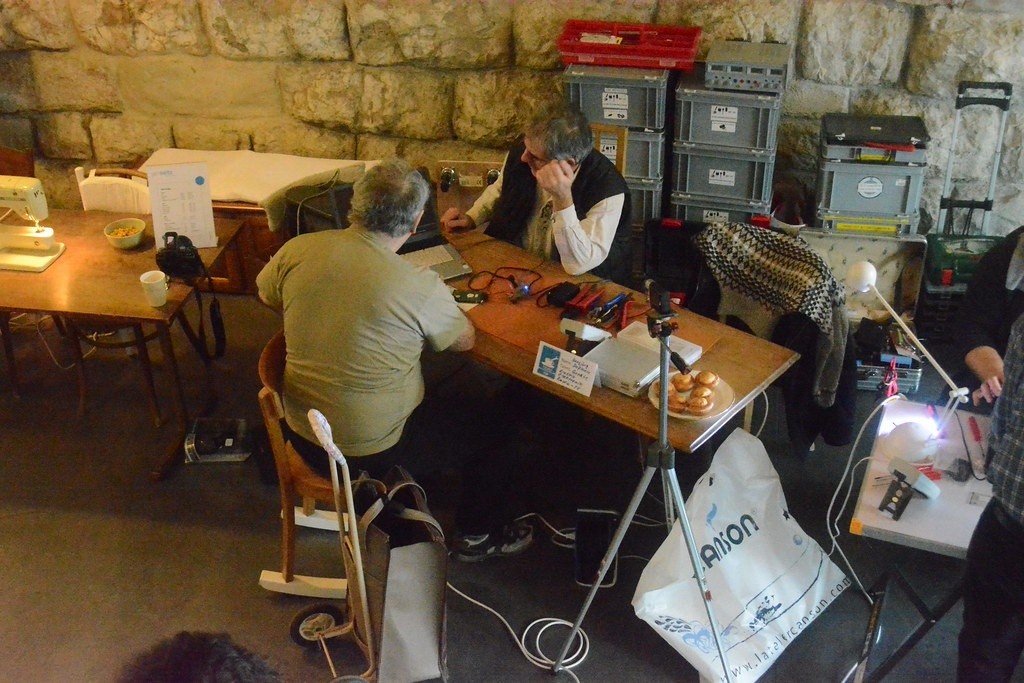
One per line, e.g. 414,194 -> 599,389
60,166 -> 165,431
254,327 -> 408,602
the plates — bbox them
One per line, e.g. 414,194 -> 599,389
648,369 -> 735,421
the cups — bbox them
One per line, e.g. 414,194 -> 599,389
140,270 -> 167,307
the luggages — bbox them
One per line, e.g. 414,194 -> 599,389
916,81 -> 1016,343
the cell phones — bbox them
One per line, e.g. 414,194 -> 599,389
452,290 -> 487,304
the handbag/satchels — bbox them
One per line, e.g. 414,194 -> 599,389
627,428 -> 855,683
345,464 -> 448,683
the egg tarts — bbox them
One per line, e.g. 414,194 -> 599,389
654,370 -> 719,415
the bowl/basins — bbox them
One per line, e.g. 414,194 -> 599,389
104,218 -> 146,249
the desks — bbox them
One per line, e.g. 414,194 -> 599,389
410,220 -> 801,457
848,398 -> 994,683
0,207 -> 246,481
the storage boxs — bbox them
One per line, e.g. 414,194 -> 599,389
817,145 -> 930,222
555,16 -> 793,293
814,206 -> 921,234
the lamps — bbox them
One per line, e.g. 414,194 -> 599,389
845,260 -> 970,463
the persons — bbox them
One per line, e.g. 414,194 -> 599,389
440,100 -> 633,284
937,225 -> 1024,416
114,633 -> 282,683
255,159 -> 539,563
957,315 -> 1024,683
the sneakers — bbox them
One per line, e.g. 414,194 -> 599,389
451,521 -> 535,565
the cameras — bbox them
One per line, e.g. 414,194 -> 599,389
155,232 -> 207,285
643,218 -> 710,314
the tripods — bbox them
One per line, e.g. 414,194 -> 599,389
553,315 -> 734,683
